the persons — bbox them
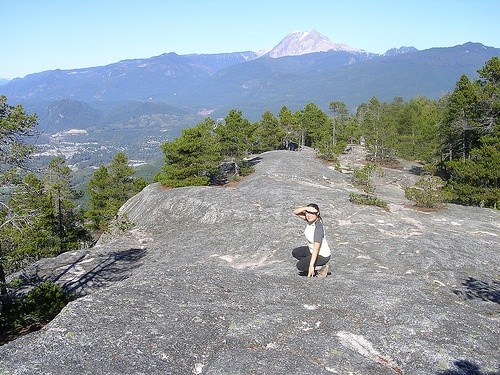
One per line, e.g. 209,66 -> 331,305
292,203 -> 331,278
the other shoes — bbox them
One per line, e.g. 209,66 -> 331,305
316,265 -> 329,278
